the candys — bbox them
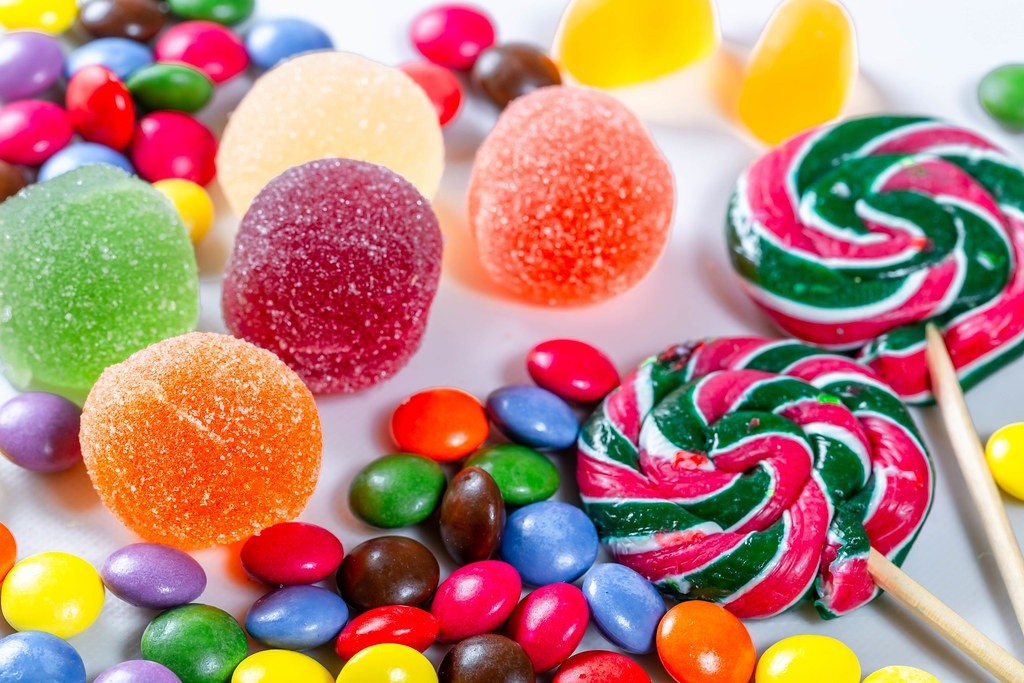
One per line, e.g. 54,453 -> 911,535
0,0 -> 1024,683
